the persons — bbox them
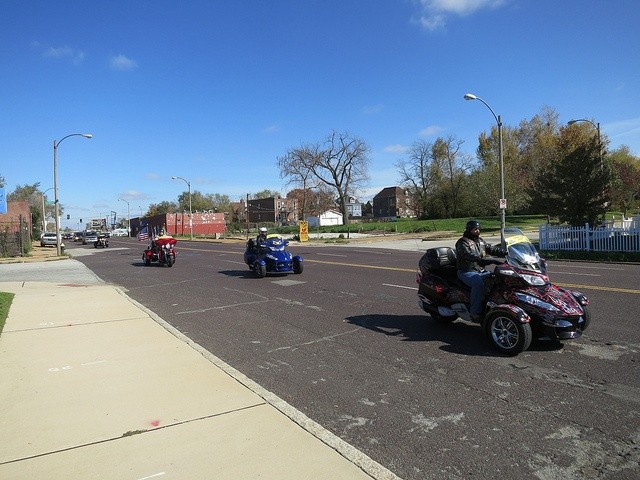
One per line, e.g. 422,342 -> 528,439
155,229 -> 167,240
455,221 -> 509,324
257,227 -> 267,248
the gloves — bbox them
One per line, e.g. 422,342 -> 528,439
478,259 -> 485,267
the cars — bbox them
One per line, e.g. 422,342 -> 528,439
41,232 -> 57,247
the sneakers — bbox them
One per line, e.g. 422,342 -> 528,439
470,312 -> 482,319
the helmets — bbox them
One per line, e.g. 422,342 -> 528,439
159,229 -> 164,236
259,227 -> 268,234
466,221 -> 480,231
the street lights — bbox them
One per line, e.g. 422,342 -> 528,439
171,176 -> 193,240
40,185 -> 60,234
53,132 -> 94,257
116,197 -> 131,238
567,118 -> 607,227
462,93 -> 508,250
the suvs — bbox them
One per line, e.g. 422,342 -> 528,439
73,232 -> 83,241
82,232 -> 99,245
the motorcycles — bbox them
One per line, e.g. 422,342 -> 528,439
416,227 -> 591,354
94,235 -> 110,248
142,237 -> 177,268
244,237 -> 304,278
